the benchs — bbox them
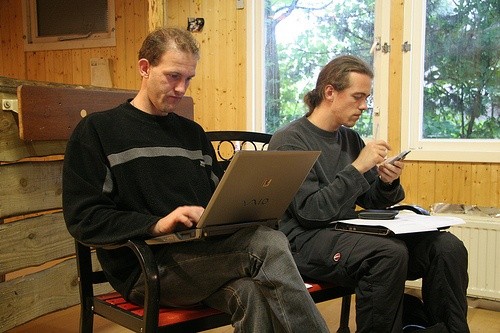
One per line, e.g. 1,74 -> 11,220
73,131 -> 431,332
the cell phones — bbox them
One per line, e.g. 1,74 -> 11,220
388,149 -> 411,165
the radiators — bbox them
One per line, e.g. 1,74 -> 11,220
405,212 -> 500,301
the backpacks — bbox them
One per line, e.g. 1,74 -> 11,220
401,293 -> 431,333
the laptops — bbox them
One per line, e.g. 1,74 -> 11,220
334,223 -> 451,234
145,149 -> 322,244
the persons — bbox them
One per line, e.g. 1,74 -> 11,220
267,57 -> 469,333
62,27 -> 330,333
